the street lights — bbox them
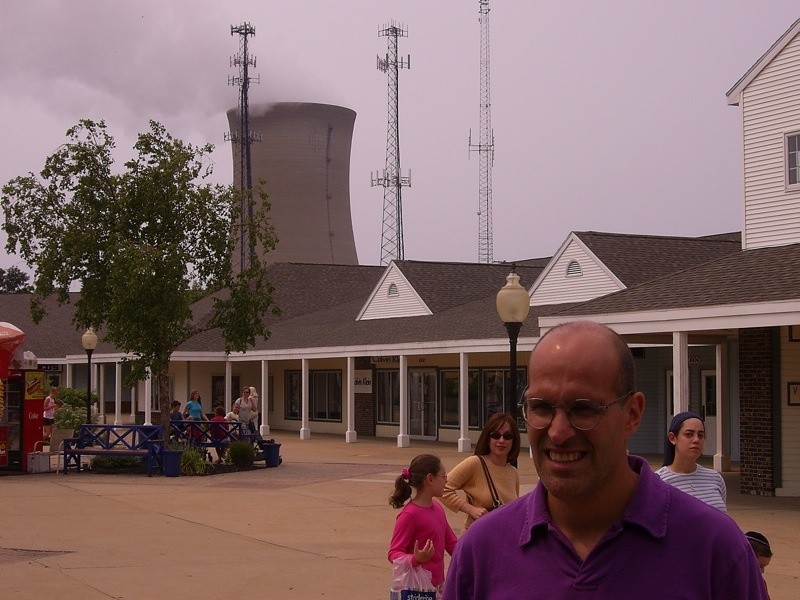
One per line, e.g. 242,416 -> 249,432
495,261 -> 531,423
81,326 -> 98,445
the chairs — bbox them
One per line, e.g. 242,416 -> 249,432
33,428 -> 75,475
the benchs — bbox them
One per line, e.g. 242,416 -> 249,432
64,420 -> 258,476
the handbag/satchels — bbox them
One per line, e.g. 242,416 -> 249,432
390,554 -> 442,600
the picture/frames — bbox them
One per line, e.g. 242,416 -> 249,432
787,382 -> 800,406
788,325 -> 800,342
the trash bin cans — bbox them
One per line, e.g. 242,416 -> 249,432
163,450 -> 183,477
262,443 -> 281,468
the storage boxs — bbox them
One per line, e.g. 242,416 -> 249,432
27,454 -> 64,473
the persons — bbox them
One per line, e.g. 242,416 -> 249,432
744,531 -> 772,575
170,386 -> 258,464
655,412 -> 727,516
436,318 -> 767,600
437,412 -> 520,536
387,454 -> 459,596
43,387 -> 59,440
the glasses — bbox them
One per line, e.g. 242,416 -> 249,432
517,384 -> 635,431
432,471 -> 448,481
489,431 -> 515,440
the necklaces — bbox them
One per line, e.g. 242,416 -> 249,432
685,477 -> 695,491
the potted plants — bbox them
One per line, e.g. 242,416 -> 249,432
162,439 -> 184,476
